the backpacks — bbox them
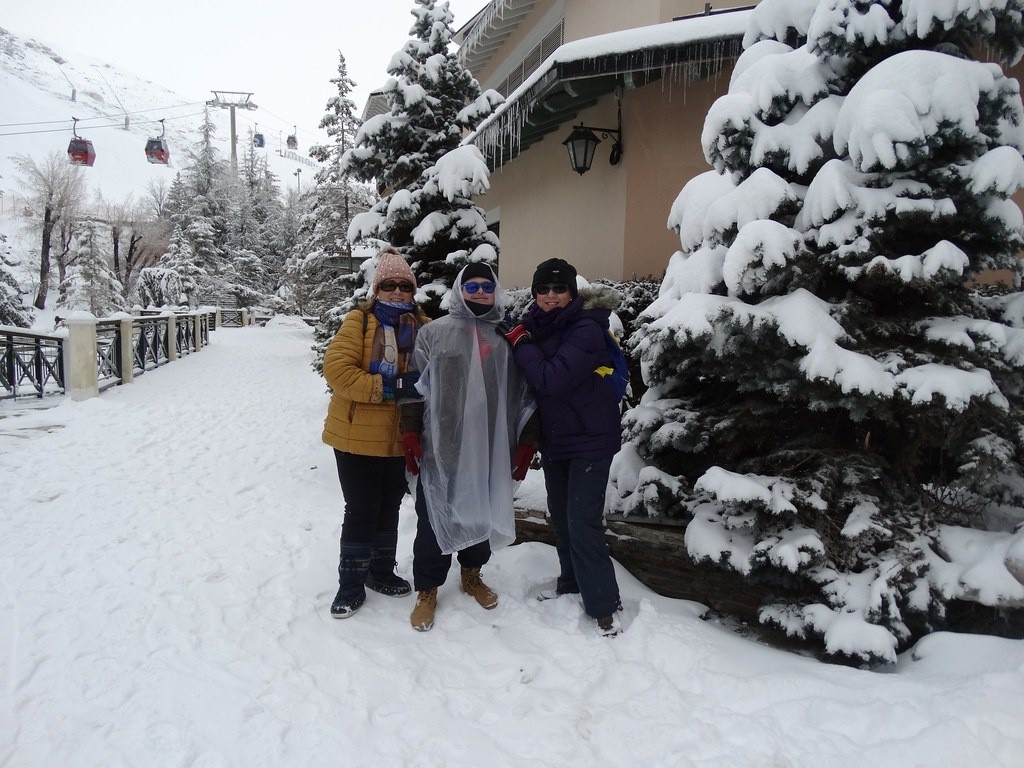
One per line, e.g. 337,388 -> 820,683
602,324 -> 629,403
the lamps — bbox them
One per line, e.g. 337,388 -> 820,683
562,121 -> 623,176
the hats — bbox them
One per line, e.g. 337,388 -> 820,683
461,262 -> 495,284
531,258 -> 577,298
372,246 -> 417,296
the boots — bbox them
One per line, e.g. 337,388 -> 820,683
461,566 -> 498,609
332,539 -> 371,618
411,586 -> 437,630
368,532 -> 412,598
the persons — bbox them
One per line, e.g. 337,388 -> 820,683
397,262 -> 537,633
322,247 -> 432,618
495,258 -> 630,638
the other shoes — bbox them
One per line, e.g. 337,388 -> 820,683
537,578 -> 579,601
594,606 -> 618,637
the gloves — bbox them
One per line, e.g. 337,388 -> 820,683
495,317 -> 531,347
391,369 -> 423,400
402,431 -> 423,475
511,440 -> 536,482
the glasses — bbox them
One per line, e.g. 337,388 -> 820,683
535,284 -> 570,294
461,281 -> 497,294
377,280 -> 415,292
